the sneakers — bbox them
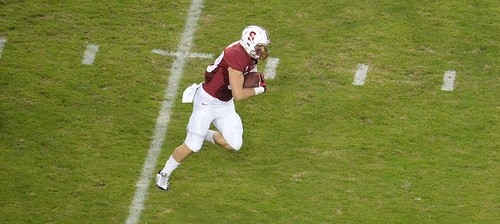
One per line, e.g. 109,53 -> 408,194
155,170 -> 170,190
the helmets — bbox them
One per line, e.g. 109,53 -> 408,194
239,25 -> 269,60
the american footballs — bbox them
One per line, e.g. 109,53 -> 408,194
243,72 -> 264,88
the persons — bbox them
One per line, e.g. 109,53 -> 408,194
155,25 -> 270,191
